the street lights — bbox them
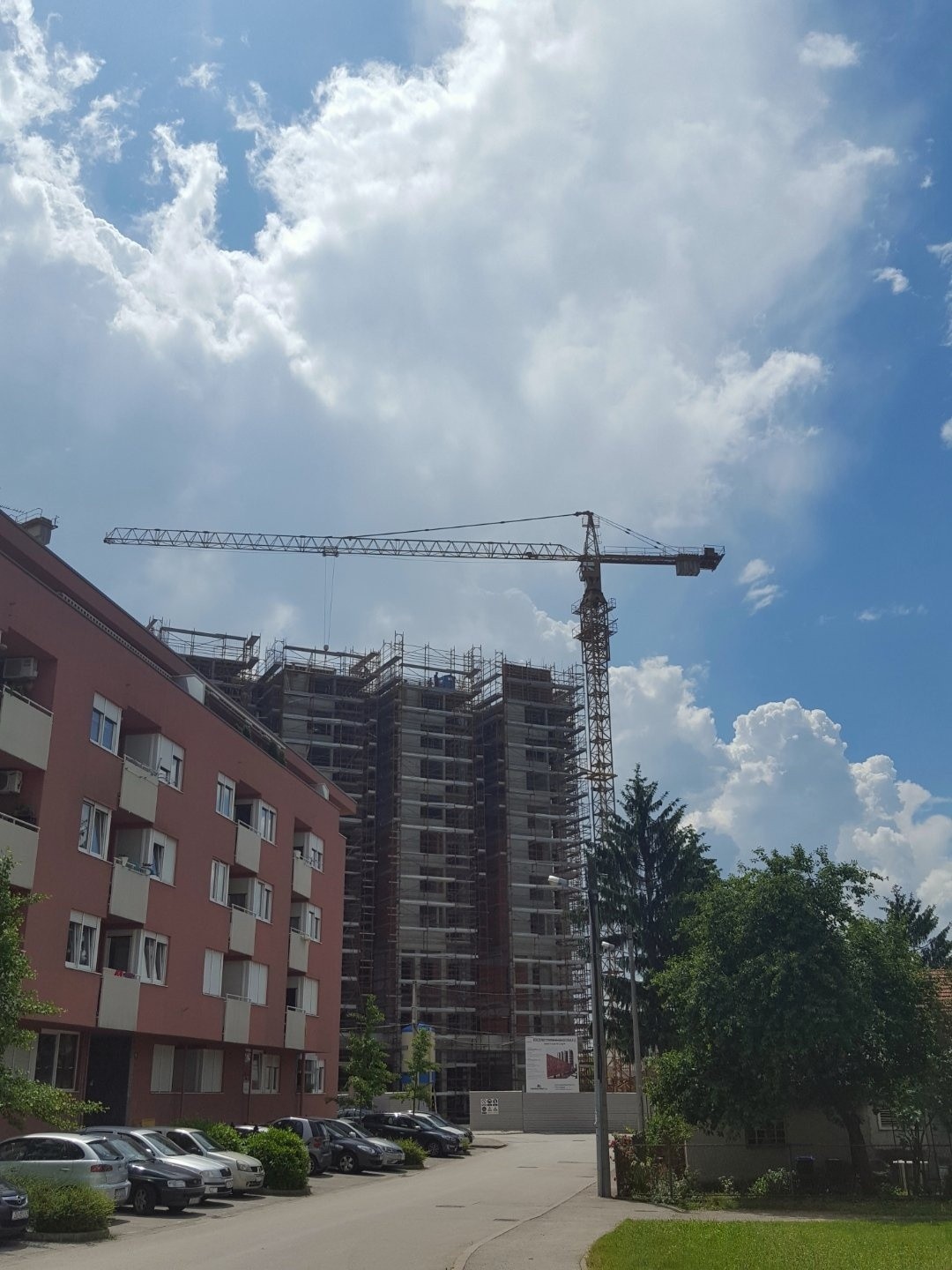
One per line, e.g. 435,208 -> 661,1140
548,852 -> 611,1198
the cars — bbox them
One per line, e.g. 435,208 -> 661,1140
81,1132 -> 206,1217
77,1125 -> 233,1206
231,1106 -> 475,1179
141,1126 -> 266,1197
0,1132 -> 132,1223
0,1177 -> 31,1241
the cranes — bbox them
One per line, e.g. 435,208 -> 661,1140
99,509 -> 730,1091
